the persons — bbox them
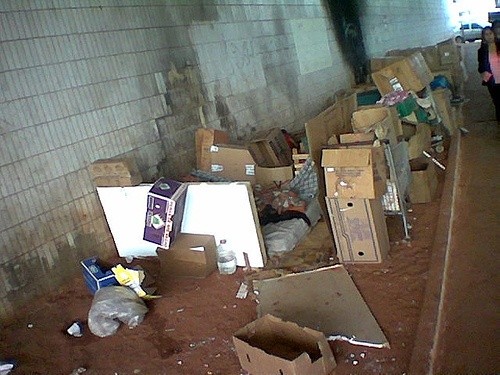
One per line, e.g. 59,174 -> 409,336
478,20 -> 500,124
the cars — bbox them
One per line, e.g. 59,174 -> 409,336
452,22 -> 484,43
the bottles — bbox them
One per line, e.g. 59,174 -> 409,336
216,240 -> 237,275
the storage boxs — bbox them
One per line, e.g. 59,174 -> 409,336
82,38 -> 466,293
232,313 -> 338,375
